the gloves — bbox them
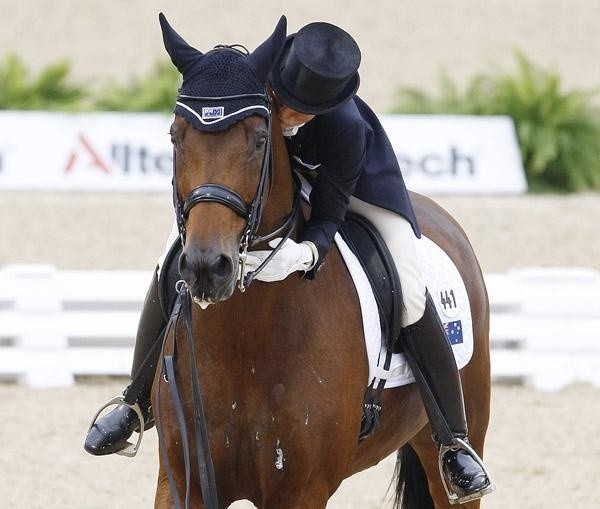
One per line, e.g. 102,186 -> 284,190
238,237 -> 313,283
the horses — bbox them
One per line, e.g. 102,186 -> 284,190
150,11 -> 491,509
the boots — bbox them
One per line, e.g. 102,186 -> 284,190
83,265 -> 167,455
404,289 -> 486,495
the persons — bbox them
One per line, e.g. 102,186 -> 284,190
84,21 -> 495,503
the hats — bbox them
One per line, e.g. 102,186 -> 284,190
269,21 -> 360,115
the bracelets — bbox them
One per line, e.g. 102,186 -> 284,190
302,241 -> 319,271
